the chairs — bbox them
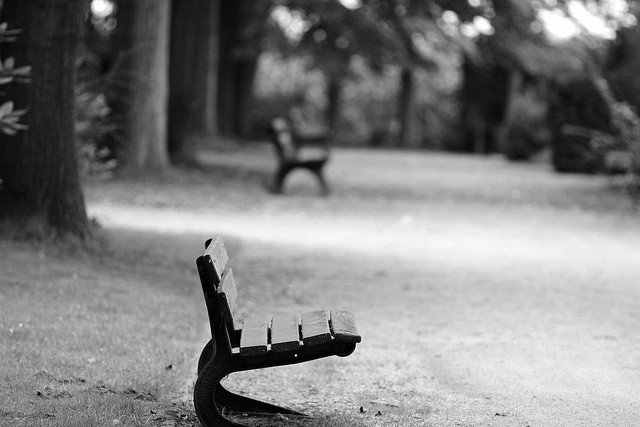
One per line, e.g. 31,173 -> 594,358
193,232 -> 362,425
268,114 -> 331,197
288,105 -> 335,149
